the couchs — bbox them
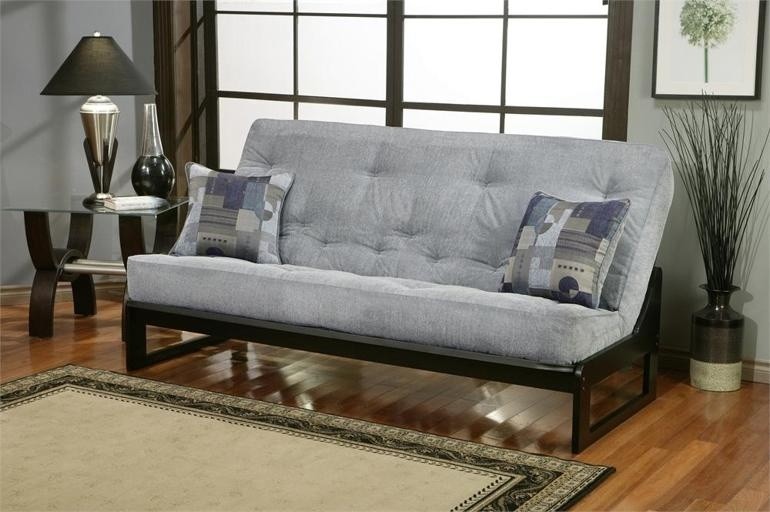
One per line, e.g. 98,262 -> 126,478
117,119 -> 675,454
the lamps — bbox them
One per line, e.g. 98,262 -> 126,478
41,32 -> 158,201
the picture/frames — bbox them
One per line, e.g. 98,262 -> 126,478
650,0 -> 764,100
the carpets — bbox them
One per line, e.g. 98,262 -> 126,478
0,364 -> 615,512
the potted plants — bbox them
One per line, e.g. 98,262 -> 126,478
659,91 -> 769,392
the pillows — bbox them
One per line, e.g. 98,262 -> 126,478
499,191 -> 631,308
169,161 -> 295,266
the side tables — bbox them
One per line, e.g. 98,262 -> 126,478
1,195 -> 192,339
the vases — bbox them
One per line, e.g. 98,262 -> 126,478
131,104 -> 174,200
